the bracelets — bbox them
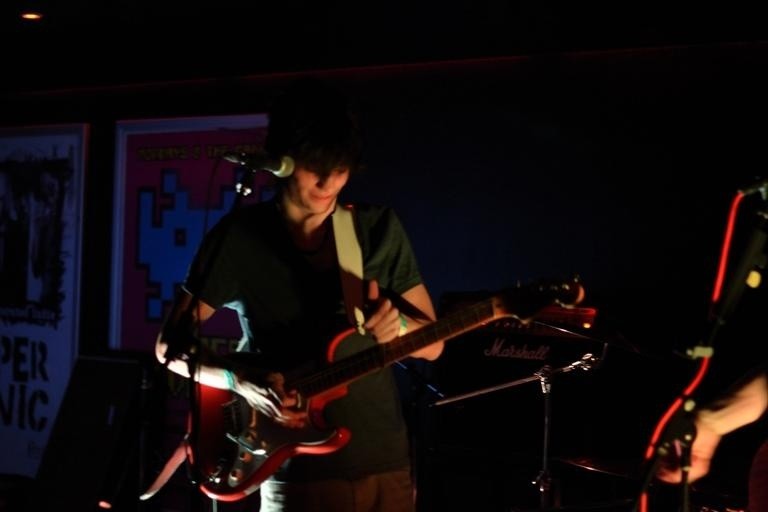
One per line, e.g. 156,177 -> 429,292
398,312 -> 408,337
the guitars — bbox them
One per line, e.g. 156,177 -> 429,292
186,276 -> 585,501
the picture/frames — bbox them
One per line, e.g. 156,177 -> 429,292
109,115 -> 268,349
3,121 -> 86,478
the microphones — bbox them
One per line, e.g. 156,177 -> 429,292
223,151 -> 296,178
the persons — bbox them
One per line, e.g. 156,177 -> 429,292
154,107 -> 445,512
651,365 -> 768,484
